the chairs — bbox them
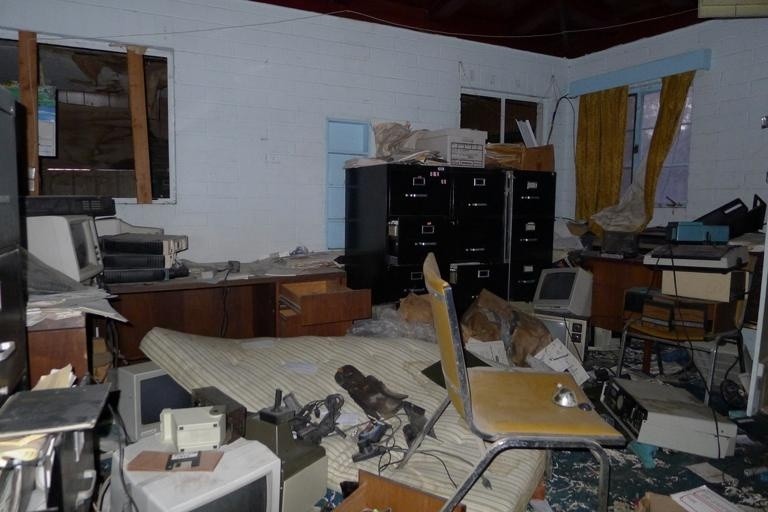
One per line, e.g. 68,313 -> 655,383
395,249 -> 627,511
618,269 -> 752,407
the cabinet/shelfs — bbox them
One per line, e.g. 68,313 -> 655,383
346,160 -> 558,310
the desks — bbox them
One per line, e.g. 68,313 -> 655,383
107,261 -> 373,366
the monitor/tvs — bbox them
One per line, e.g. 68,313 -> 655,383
109,431 -> 284,512
532,267 -> 593,318
531,313 -> 588,364
115,361 -> 190,442
23,213 -> 106,283
